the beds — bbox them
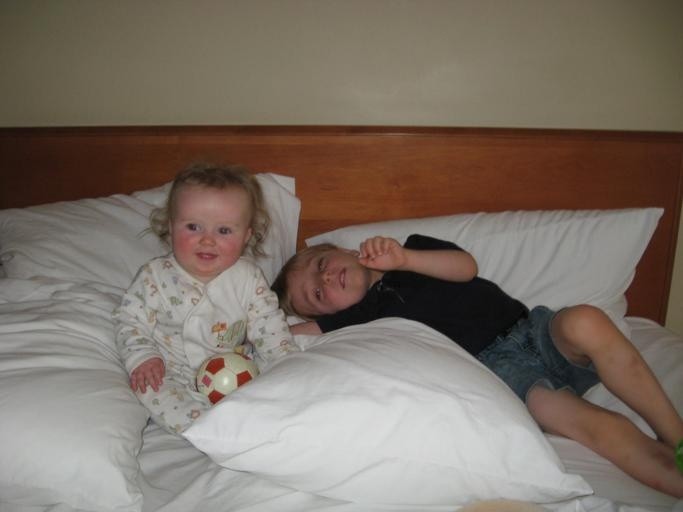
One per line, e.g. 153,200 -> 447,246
0,125 -> 683,512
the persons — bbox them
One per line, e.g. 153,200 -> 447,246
270,232 -> 683,499
112,161 -> 304,439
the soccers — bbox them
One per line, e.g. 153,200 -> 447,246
196,351 -> 259,403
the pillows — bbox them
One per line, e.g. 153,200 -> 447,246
0,169 -> 667,512
